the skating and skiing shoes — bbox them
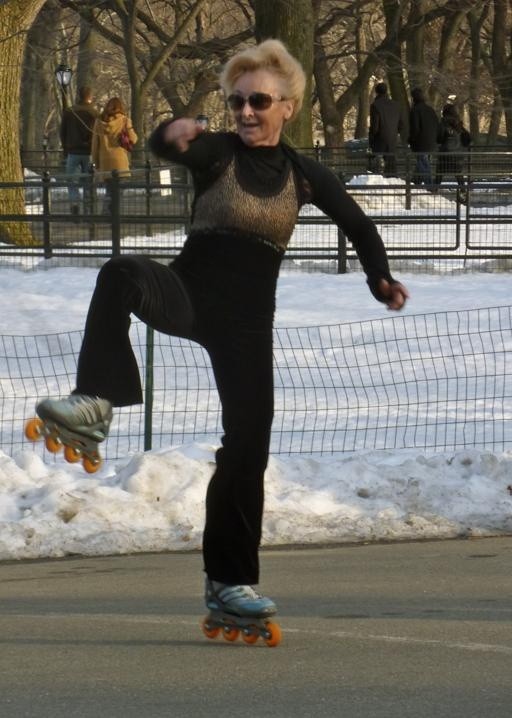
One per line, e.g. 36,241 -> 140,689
26,391 -> 113,473
202,576 -> 282,647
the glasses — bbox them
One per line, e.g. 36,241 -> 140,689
226,92 -> 291,111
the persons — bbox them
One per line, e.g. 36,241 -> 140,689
36,38 -> 412,619
368,81 -> 410,178
432,103 -> 470,202
408,86 -> 442,193
91,95 -> 138,228
58,83 -> 98,223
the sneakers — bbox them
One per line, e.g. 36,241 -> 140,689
72,203 -> 81,224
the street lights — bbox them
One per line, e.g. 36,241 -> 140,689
54,63 -> 73,164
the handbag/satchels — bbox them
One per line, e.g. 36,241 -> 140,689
461,129 -> 472,147
121,129 -> 133,152
